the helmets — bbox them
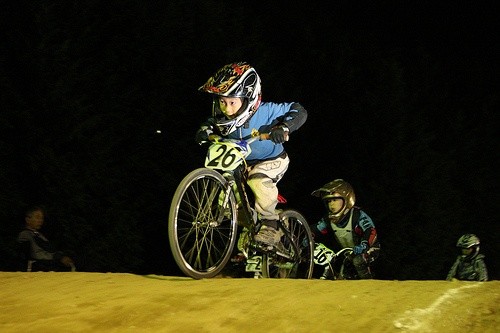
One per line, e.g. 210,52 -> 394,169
311,180 -> 355,220
456,234 -> 480,262
198,62 -> 262,135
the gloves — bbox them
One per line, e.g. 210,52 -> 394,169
195,129 -> 208,144
353,245 -> 363,254
269,125 -> 288,144
303,238 -> 308,247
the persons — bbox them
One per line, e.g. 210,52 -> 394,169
302,179 -> 377,280
446,234 -> 488,282
18,208 -> 73,272
196,63 -> 307,263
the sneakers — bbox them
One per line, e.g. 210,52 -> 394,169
254,226 -> 284,246
237,227 -> 251,251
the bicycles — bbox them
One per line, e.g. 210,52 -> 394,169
311,236 -> 381,280
167,119 -> 315,281
